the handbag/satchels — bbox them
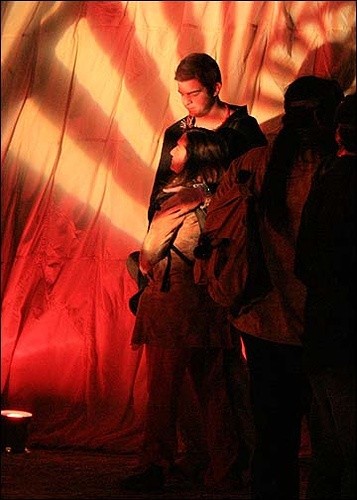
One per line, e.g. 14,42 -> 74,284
192,148 -> 270,314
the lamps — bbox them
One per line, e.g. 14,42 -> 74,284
0,410 -> 33,454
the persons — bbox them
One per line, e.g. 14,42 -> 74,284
143,52 -> 270,477
121,125 -> 242,490
193,76 -> 357,500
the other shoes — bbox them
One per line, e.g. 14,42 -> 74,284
121,472 -> 164,491
